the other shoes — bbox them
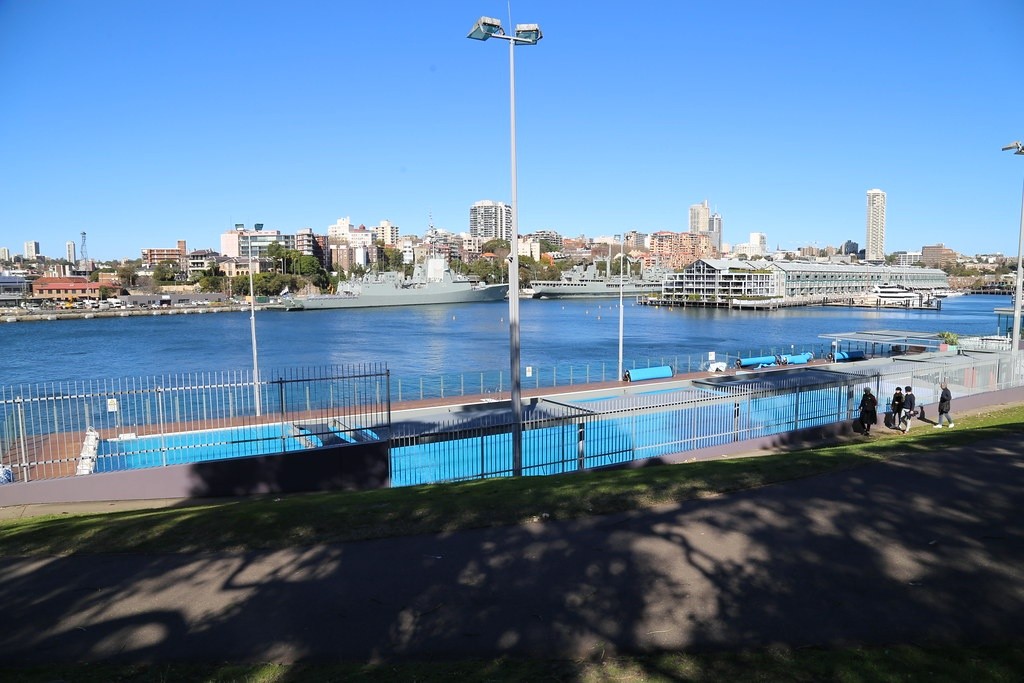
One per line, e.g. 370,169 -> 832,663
933,424 -> 942,429
863,430 -> 870,436
903,431 -> 908,434
948,423 -> 954,429
890,425 -> 901,430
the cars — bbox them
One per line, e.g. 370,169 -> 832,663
142,305 -> 148,308
99,301 -> 109,307
91,301 -> 98,307
127,303 -> 134,308
77,302 -> 84,308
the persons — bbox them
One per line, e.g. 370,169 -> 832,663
933,381 -> 954,430
858,387 -> 878,436
890,386 -> 915,436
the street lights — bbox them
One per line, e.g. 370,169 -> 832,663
467,17 -> 543,476
1001,142 -> 1024,375
235,223 -> 264,416
615,230 -> 636,382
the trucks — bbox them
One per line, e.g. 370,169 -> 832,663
108,299 -> 121,307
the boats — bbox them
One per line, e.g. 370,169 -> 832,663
859,282 -> 936,304
1011,293 -> 1024,307
280,225 -> 509,309
530,259 -> 667,298
